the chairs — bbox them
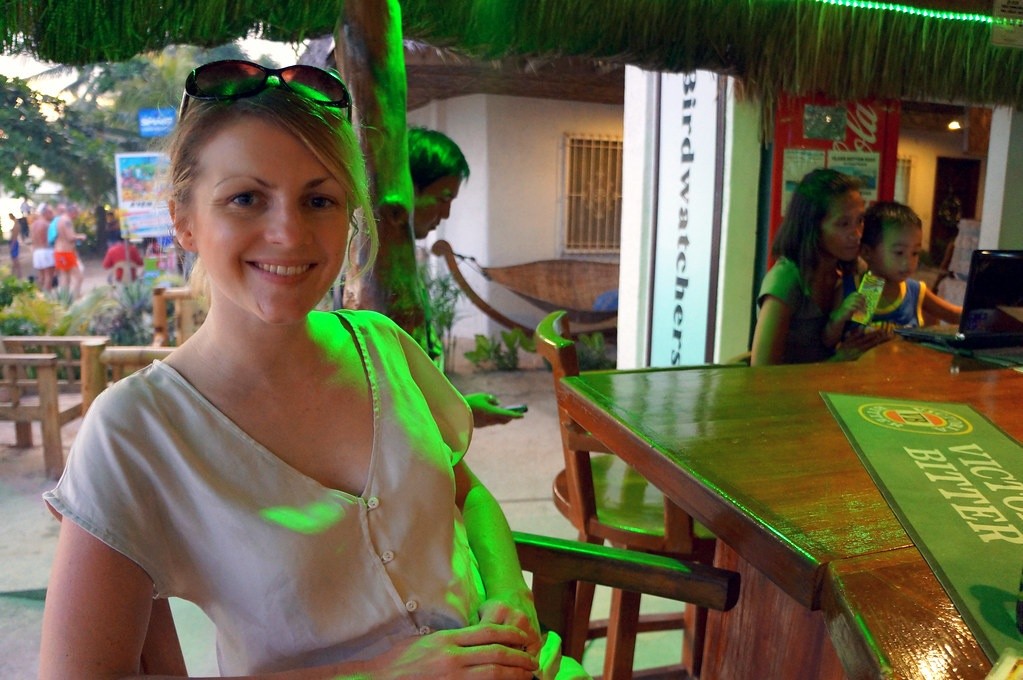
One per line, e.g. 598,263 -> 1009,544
134,531 -> 744,680
0,333 -> 114,482
532,309 -> 720,680
81,284 -> 213,421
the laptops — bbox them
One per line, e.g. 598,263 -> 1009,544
893,250 -> 1023,349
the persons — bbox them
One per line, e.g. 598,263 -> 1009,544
824,200 -> 962,349
7,197 -> 165,302
750,168 -> 893,367
333,127 -> 525,429
37,61 -> 542,680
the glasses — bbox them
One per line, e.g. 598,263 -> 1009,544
178,59 -> 354,132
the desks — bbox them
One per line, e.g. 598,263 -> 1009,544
558,321 -> 1023,680
